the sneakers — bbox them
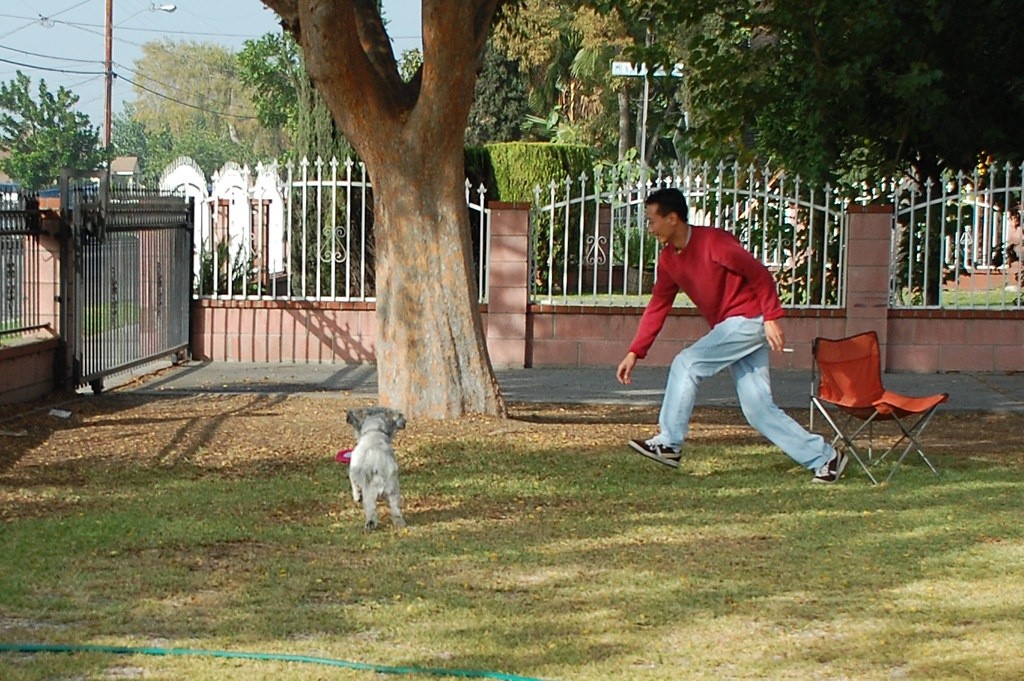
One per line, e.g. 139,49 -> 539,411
627,435 -> 682,467
811,447 -> 848,484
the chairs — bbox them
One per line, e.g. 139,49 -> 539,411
806,331 -> 950,485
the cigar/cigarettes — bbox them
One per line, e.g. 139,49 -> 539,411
782,347 -> 794,354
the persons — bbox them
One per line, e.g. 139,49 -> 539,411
617,190 -> 850,483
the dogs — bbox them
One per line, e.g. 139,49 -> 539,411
346,406 -> 410,531
